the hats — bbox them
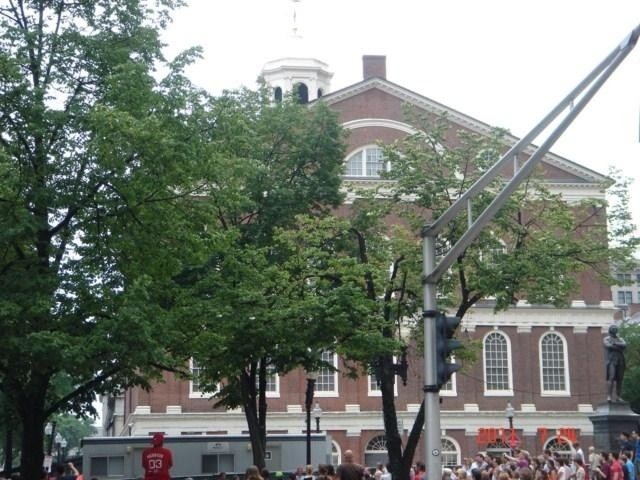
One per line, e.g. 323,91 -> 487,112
152,433 -> 165,446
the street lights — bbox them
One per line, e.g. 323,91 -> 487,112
45,423 -> 54,455
503,400 -> 516,456
54,432 -> 62,461
62,439 -> 68,460
312,402 -> 323,435
303,367 -> 323,473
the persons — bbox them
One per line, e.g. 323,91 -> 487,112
603,325 -> 627,404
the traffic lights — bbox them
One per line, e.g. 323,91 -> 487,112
436,313 -> 463,387
